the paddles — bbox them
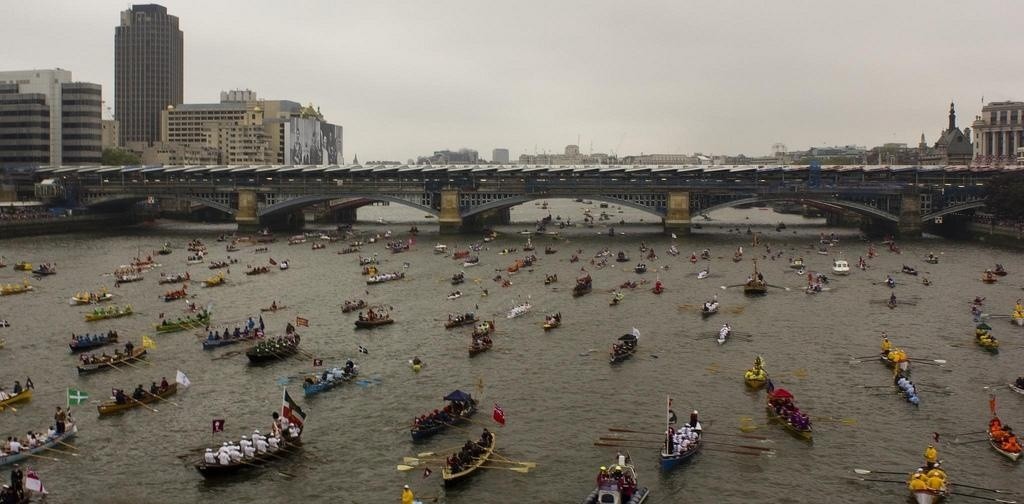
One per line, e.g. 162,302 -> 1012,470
981,313 -> 1011,319
37,444 -> 80,456
288,370 -> 322,380
854,468 -> 909,475
128,396 -> 160,412
766,284 -> 791,292
397,451 -> 451,471
908,357 -> 946,364
873,281 -> 885,285
145,391 -> 178,406
1,401 -> 16,413
464,452 -> 537,473
593,428 -> 665,450
349,378 -> 369,388
19,450 -> 59,461
720,284 -> 745,290
270,344 -> 315,366
852,355 -> 882,363
237,460 -> 293,478
949,482 -> 1018,504
698,431 -> 769,456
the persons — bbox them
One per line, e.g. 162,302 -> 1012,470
1,217 -> 419,502
414,217 -> 1023,502
291,129 -> 338,165
